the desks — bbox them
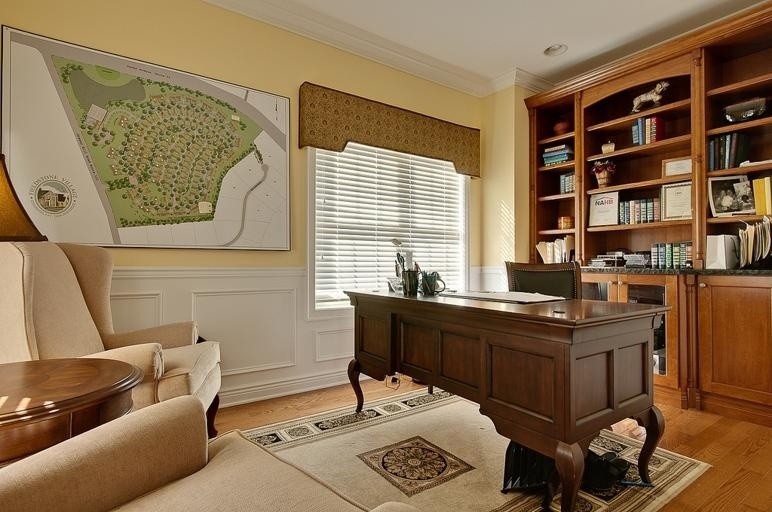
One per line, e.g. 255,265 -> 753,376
344,289 -> 667,512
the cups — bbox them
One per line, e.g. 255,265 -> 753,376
422,276 -> 436,295
401,271 -> 419,298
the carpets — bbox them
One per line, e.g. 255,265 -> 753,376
242,384 -> 712,512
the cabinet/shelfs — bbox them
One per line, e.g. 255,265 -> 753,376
522,2 -> 771,427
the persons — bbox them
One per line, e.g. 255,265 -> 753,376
715,188 -> 753,212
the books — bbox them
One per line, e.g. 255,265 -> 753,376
587,240 -> 694,269
631,116 -> 665,146
708,132 -> 751,172
560,171 -> 576,194
542,144 -> 574,167
535,234 -> 575,264
619,198 -> 660,225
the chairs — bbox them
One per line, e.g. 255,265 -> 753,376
0,237 -> 222,438
505,258 -> 582,300
0,393 -> 418,512
